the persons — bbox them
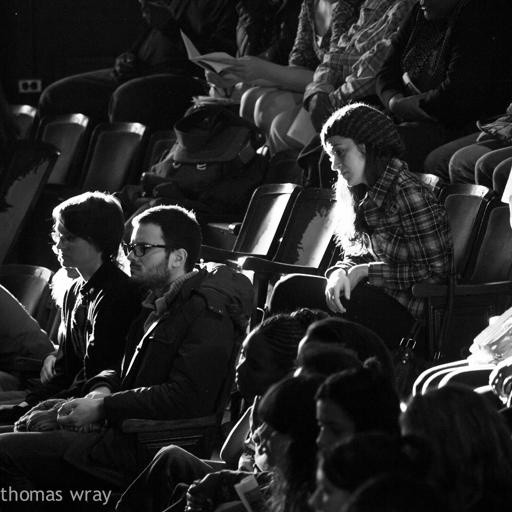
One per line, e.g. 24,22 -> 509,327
112,1 -> 510,512
35,0 -> 240,125
103,305 -> 321,511
1,203 -> 256,512
0,186 -> 142,436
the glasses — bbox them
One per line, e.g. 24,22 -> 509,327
122,242 -> 168,257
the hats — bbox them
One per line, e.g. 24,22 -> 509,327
256,311 -> 488,493
172,104 -> 253,164
320,101 -> 408,157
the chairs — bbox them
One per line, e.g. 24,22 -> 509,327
0,100 -> 512,463
